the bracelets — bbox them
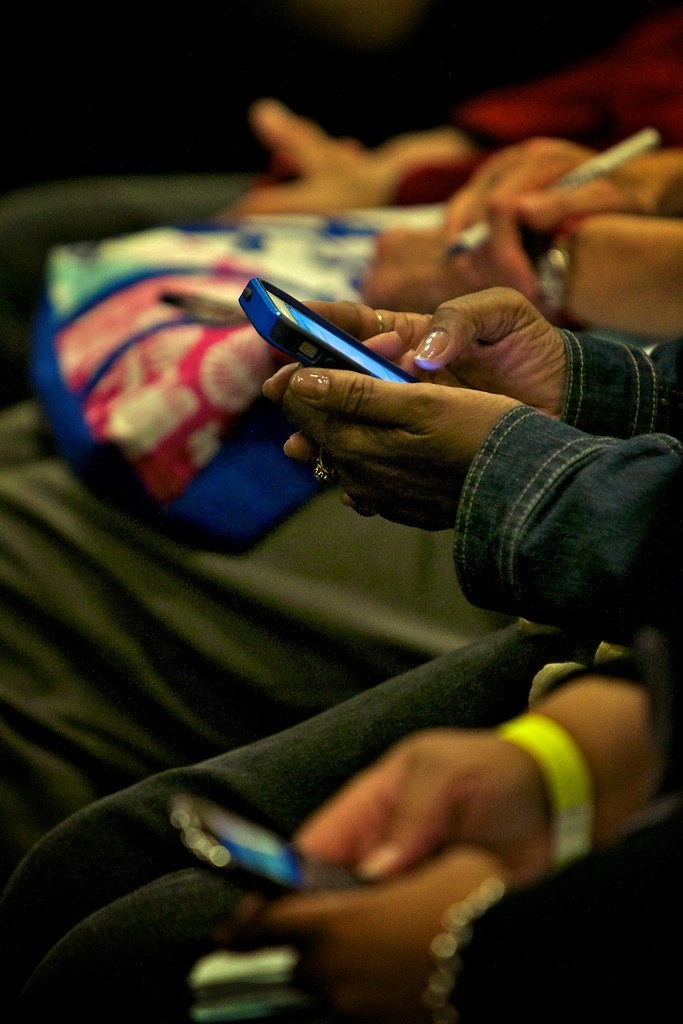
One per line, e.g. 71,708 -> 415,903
418,879 -> 516,1023
498,715 -> 596,866
527,215 -> 586,324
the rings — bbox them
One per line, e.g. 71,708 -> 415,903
375,309 -> 384,335
314,447 -> 333,482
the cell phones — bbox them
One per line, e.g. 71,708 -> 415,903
238,277 -> 423,384
168,789 -> 304,899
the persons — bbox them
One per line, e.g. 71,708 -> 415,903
223,638 -> 683,1023
217,0 -> 682,309
0,285 -> 683,1023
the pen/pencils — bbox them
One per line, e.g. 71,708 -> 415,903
444,126 -> 663,266
